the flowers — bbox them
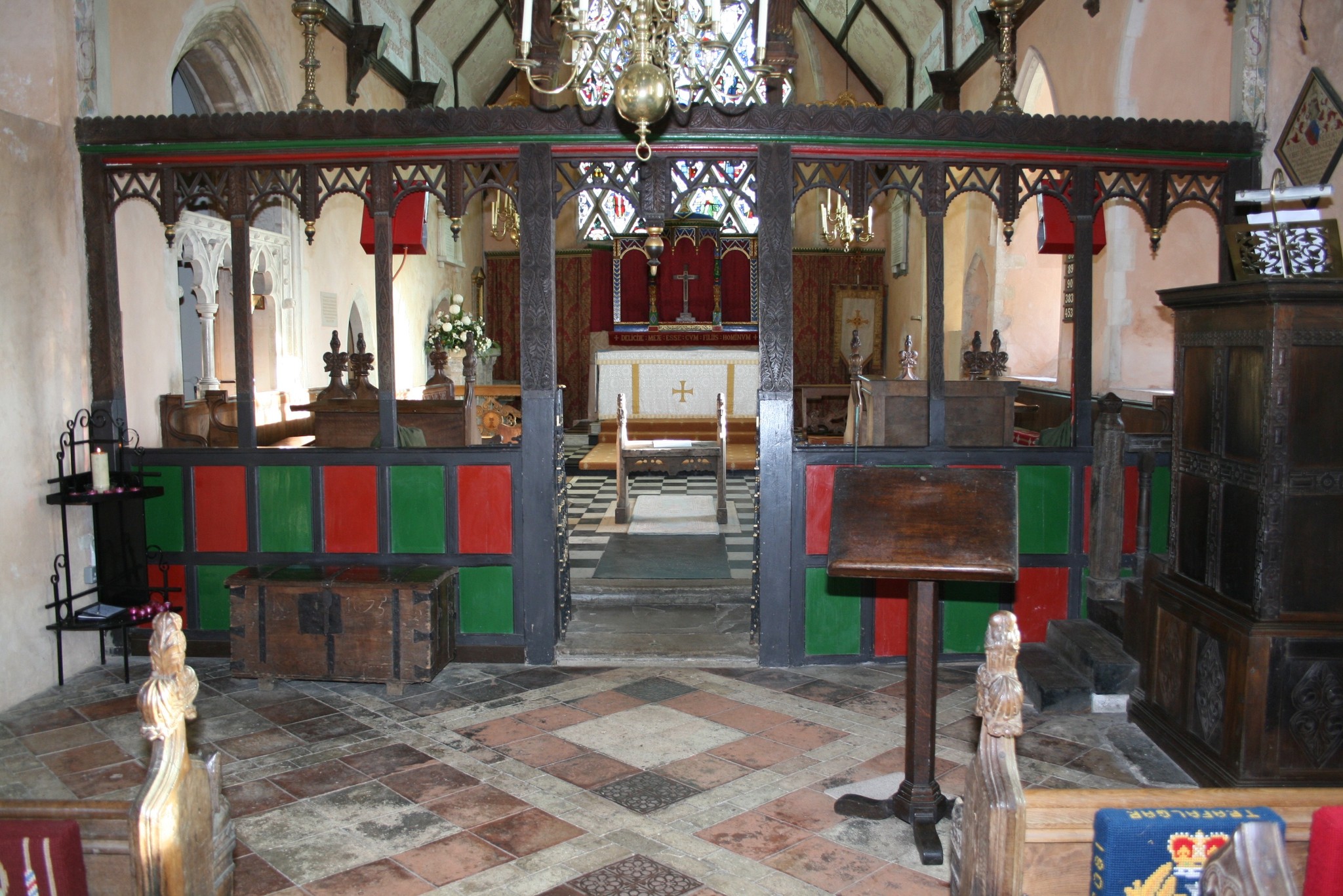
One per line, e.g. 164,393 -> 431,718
424,288 -> 501,365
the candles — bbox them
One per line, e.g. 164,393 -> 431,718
91,447 -> 110,494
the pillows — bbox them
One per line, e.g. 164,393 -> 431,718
1089,807 -> 1343,896
0,820 -> 89,896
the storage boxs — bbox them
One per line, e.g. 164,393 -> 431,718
223,566 -> 460,695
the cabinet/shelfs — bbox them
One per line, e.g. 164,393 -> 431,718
45,408 -> 183,685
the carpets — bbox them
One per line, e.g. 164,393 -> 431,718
627,495 -> 719,535
592,533 -> 732,579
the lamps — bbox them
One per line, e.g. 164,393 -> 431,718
507,0 -> 777,161
821,184 -> 874,253
1235,168 -> 1335,277
489,185 -> 520,249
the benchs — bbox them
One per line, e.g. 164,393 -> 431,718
160,329 -> 521,447
0,612 -> 236,896
1015,385 -> 1174,433
950,610 -> 1343,896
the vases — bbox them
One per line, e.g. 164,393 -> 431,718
431,348 -> 500,385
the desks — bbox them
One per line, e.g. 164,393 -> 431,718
594,351 -> 761,420
793,384 -> 851,440
